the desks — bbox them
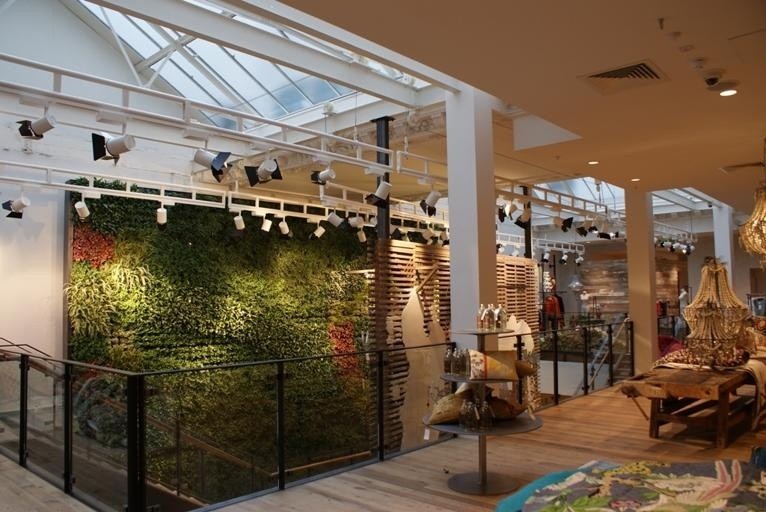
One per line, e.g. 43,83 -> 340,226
620,367 -> 758,446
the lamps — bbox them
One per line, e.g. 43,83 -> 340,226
15,97 -> 136,167
157,202 -> 171,230
498,197 -> 533,229
364,173 -> 393,207
309,154 -> 338,186
1,182 -> 31,219
733,179 -> 766,270
421,180 -> 441,218
229,208 -> 449,249
550,206 -> 626,240
542,249 -> 586,267
72,191 -> 93,220
180,128 -> 284,187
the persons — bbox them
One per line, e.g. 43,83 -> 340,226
677,288 -> 689,315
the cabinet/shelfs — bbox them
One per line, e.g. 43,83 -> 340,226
424,330 -> 544,495
